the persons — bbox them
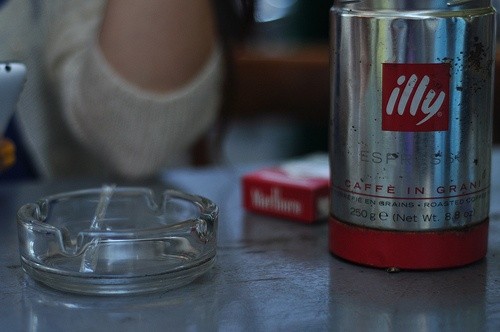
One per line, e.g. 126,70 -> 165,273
0,0 -> 222,184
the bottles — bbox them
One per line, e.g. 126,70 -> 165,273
329,0 -> 497,270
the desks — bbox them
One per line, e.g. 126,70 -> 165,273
0,143 -> 500,332
